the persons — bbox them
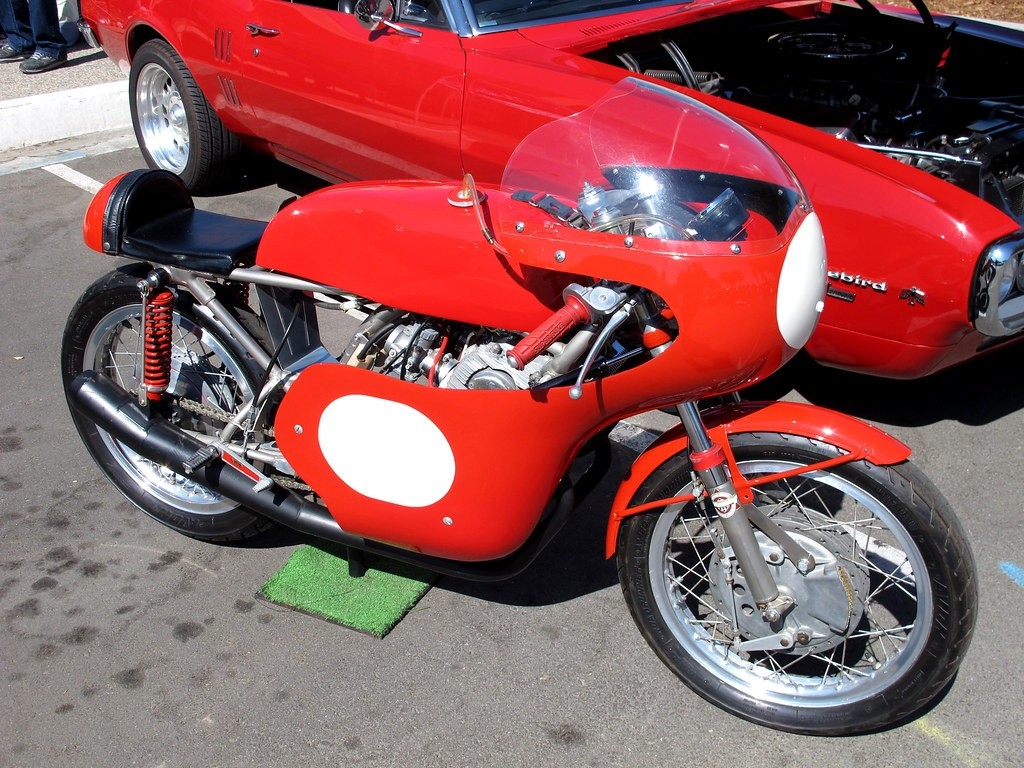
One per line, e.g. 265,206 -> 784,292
1,0 -> 67,73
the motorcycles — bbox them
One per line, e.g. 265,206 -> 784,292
61,77 -> 980,741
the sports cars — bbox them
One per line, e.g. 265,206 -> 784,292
74,2 -> 1024,383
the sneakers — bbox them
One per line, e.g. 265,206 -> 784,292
0,43 -> 33,62
19,51 -> 66,73
